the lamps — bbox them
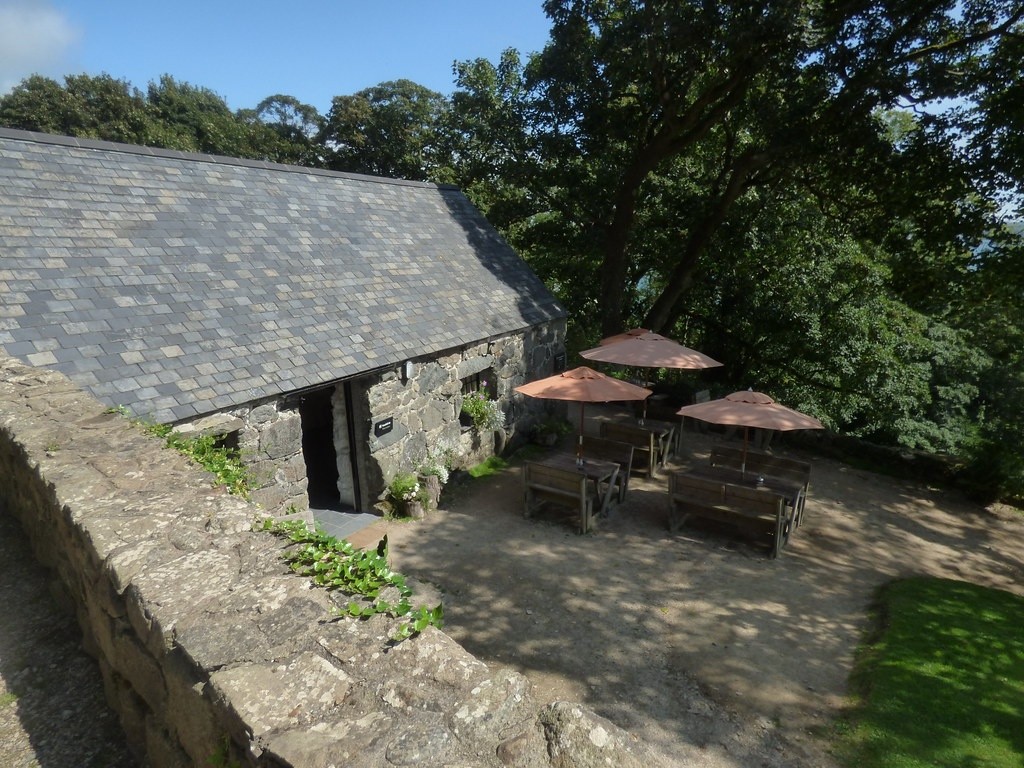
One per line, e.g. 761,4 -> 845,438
403,360 -> 414,379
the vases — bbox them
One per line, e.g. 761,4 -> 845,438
461,412 -> 473,426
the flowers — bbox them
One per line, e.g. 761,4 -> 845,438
388,473 -> 431,512
420,462 -> 449,485
461,381 -> 507,439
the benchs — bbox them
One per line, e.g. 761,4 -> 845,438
668,470 -> 789,561
568,433 -> 635,503
703,446 -> 813,525
599,420 -> 660,479
634,412 -> 685,455
522,459 -> 596,533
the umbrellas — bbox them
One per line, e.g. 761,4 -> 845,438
578,327 -> 724,425
514,367 -> 653,466
676,388 -> 826,482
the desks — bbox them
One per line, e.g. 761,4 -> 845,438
690,463 -> 805,534
616,418 -> 675,467
541,453 -> 620,516
